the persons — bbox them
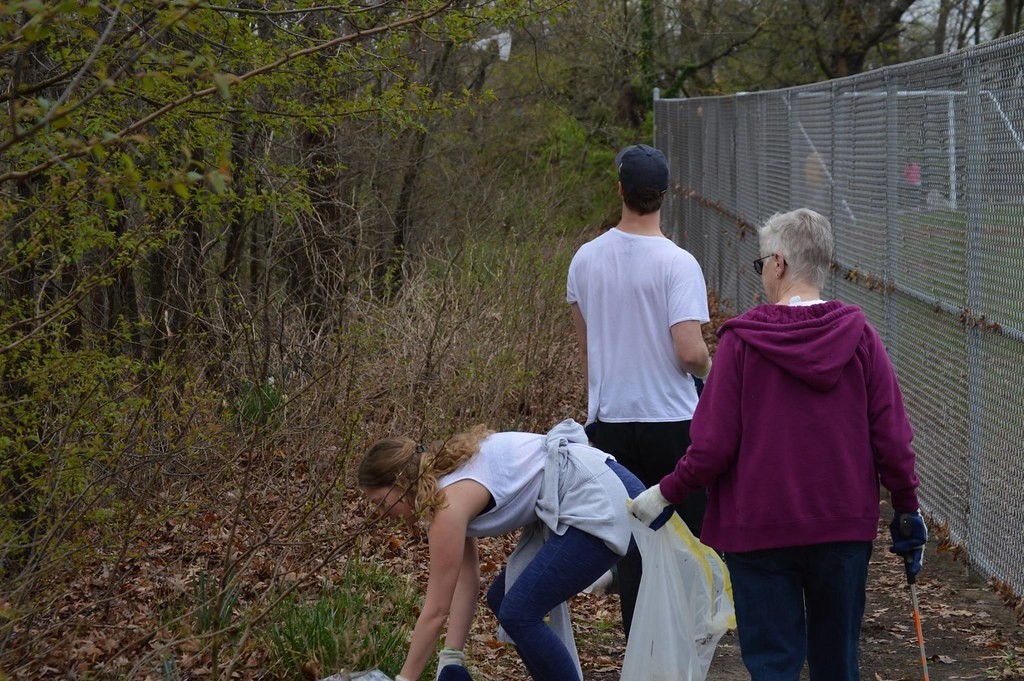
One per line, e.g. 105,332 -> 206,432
566,144 -> 713,646
358,424 -> 646,681
628,208 -> 927,681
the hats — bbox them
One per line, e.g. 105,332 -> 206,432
616,144 -> 669,199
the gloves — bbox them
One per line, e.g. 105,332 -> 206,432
435,650 -> 474,681
890,507 -> 927,584
691,358 -> 713,399
629,484 -> 671,526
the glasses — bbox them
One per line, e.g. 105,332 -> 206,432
753,254 -> 788,275
